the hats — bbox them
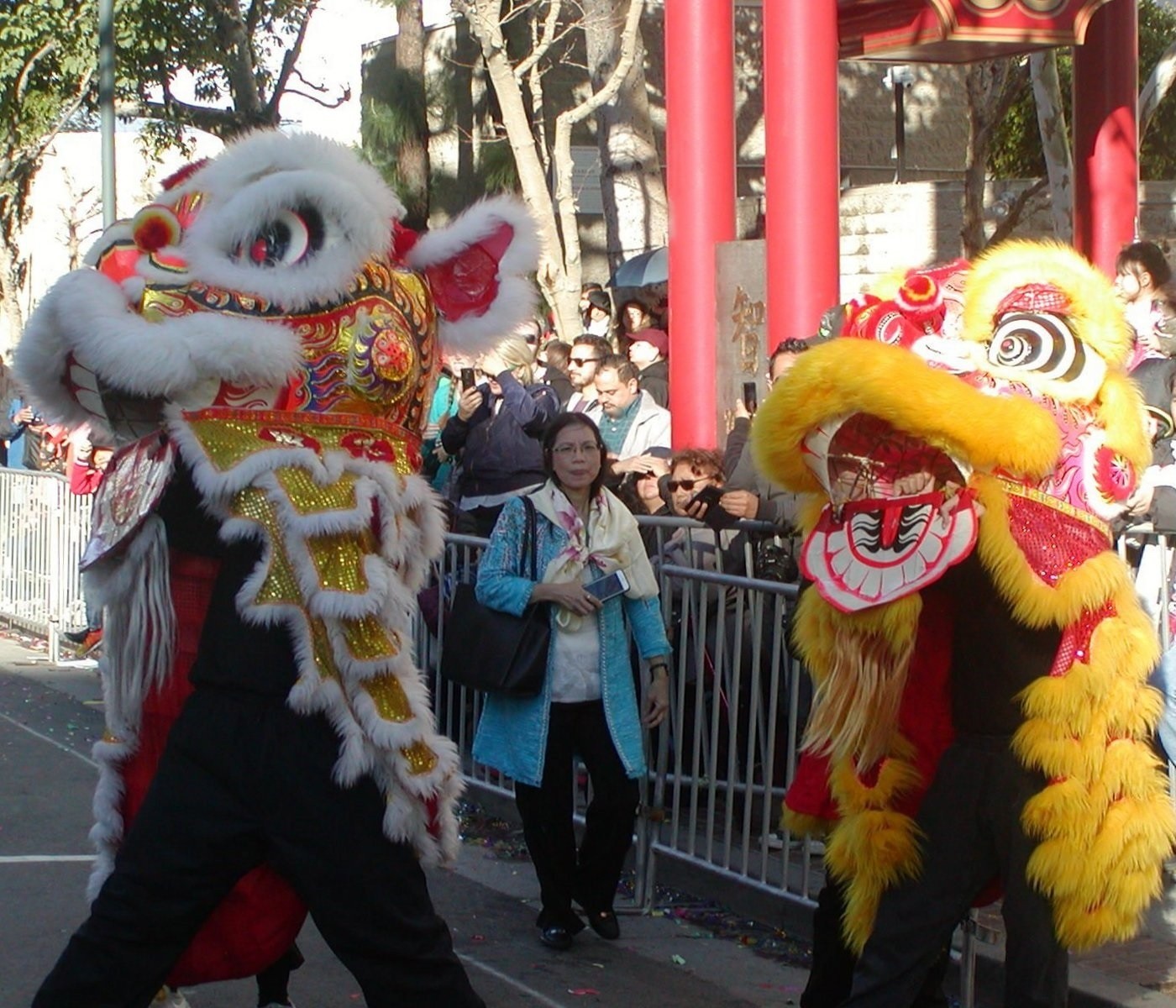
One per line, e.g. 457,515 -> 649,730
625,329 -> 668,355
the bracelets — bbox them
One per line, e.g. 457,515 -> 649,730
649,663 -> 668,671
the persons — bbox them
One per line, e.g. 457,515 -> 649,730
469,413 -> 671,946
149,941 -> 305,1008
1105,242 -> 1176,546
33,452 -> 487,1008
796,466 -> 1067,1008
0,356 -> 116,660
410,281 -> 834,861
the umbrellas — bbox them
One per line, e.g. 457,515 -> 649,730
605,248 -> 668,288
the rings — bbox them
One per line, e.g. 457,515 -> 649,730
587,594 -> 591,600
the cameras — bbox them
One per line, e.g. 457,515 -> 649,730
759,544 -> 799,585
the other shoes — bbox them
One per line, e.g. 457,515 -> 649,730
586,901 -> 620,941
542,921 -> 570,949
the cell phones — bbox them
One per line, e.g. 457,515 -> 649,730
684,486 -> 725,518
461,368 -> 475,391
581,569 -> 630,602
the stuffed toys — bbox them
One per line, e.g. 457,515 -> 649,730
10,128 -> 540,1008
747,241 -> 1176,1008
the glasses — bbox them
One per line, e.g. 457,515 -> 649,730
567,356 -> 604,367
549,440 -> 603,456
668,475 -> 715,492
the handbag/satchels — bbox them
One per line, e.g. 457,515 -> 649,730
441,493 -> 549,695
